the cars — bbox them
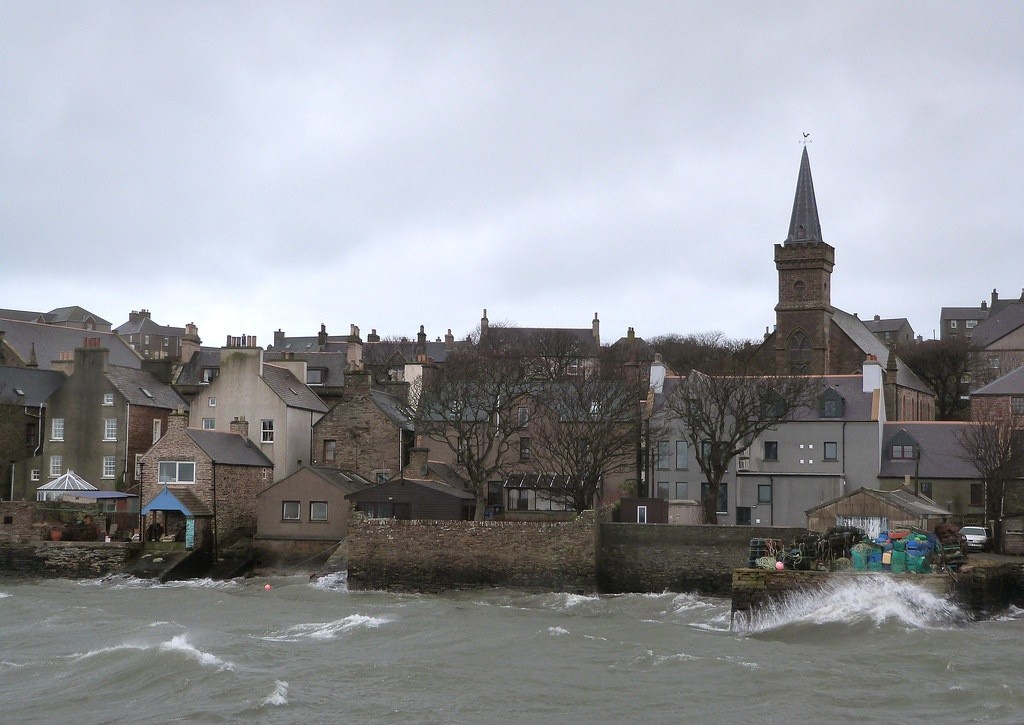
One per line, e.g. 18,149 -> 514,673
959,526 -> 991,553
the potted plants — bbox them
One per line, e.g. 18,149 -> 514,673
50,526 -> 64,541
